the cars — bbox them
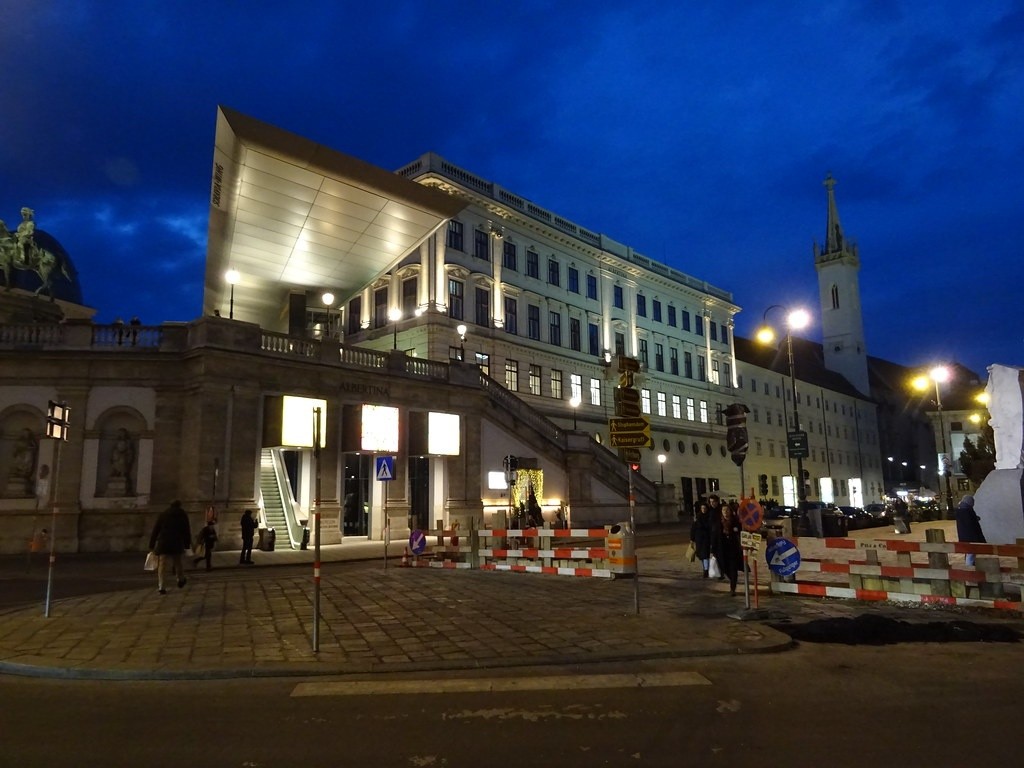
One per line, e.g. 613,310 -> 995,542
802,500 -> 893,530
758,505 -> 808,538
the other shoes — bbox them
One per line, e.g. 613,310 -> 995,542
159,590 -> 166,595
703,569 -> 708,578
730,588 -> 735,597
178,576 -> 186,589
193,559 -> 197,569
240,559 -> 256,566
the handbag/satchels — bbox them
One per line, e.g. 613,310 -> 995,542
195,539 -> 206,558
708,554 -> 722,578
685,540 -> 697,562
144,551 -> 160,570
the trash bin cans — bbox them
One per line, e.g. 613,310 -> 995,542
823,514 -> 848,537
607,522 -> 638,573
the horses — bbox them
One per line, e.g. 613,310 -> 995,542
0,219 -> 72,303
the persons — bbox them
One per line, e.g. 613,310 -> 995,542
12,428 -> 35,476
110,427 -> 132,477
112,314 -> 141,345
240,510 -> 259,564
955,495 -> 987,566
23,528 -> 48,575
14,207 -> 35,265
688,494 -> 751,595
193,521 -> 217,569
758,498 -> 778,510
148,500 -> 191,594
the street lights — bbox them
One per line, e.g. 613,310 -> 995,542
910,365 -> 958,520
758,304 -> 814,535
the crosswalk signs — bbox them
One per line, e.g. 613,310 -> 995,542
377,456 -> 394,481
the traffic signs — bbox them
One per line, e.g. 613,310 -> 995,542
620,387 -> 642,417
609,417 -> 651,448
624,448 -> 642,463
618,355 -> 641,374
